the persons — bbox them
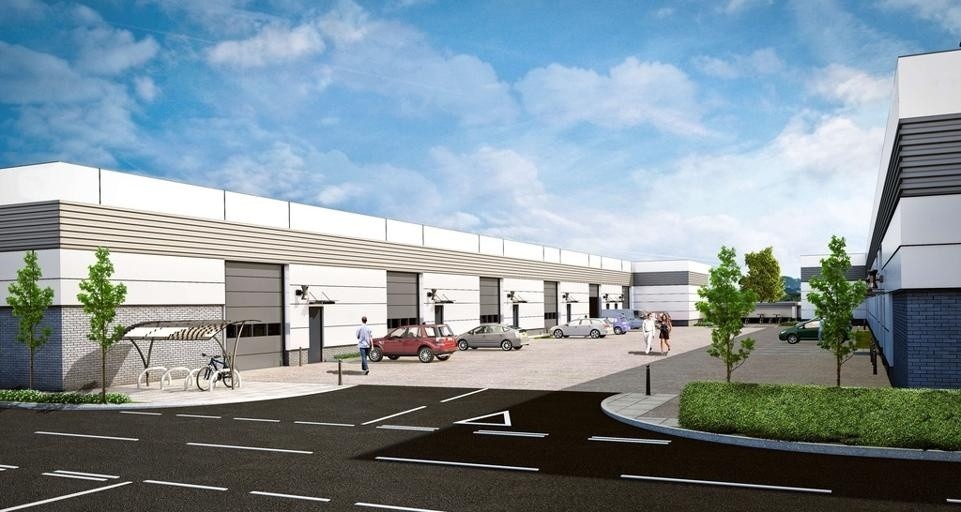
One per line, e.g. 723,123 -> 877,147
357,316 -> 375,375
641,313 -> 656,355
655,314 -> 673,354
